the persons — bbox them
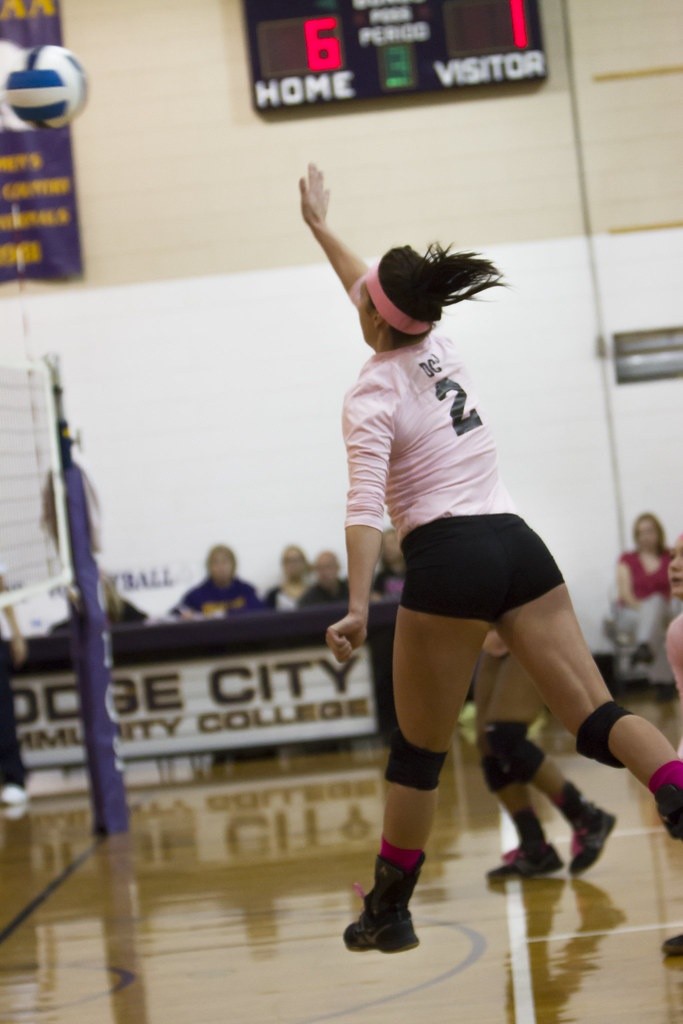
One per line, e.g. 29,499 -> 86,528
617,516 -> 683,704
296,552 -> 350,608
169,546 -> 266,622
296,163 -> 682,953
0,576 -> 31,822
661,536 -> 683,952
368,528 -> 405,604
260,546 -> 314,614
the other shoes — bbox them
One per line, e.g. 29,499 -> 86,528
630,645 -> 656,671
662,935 -> 683,955
1,784 -> 26,804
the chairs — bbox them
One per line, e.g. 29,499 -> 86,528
607,585 -> 671,679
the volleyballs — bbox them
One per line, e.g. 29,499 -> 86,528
6,44 -> 90,131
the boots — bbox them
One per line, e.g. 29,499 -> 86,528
654,784 -> 683,841
342,856 -> 422,953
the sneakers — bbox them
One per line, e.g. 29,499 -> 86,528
568,808 -> 616,873
486,844 -> 563,880
